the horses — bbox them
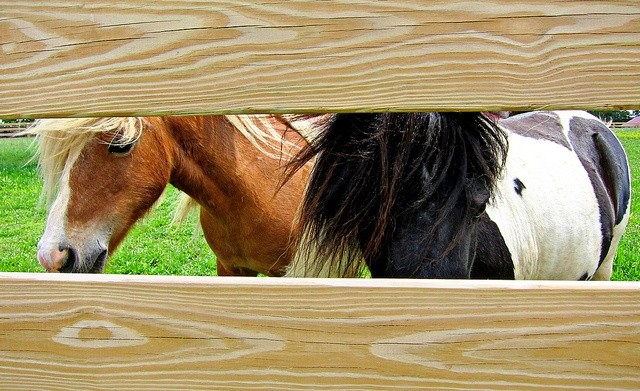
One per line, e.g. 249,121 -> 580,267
12,113 -> 371,276
269,110 -> 635,280
